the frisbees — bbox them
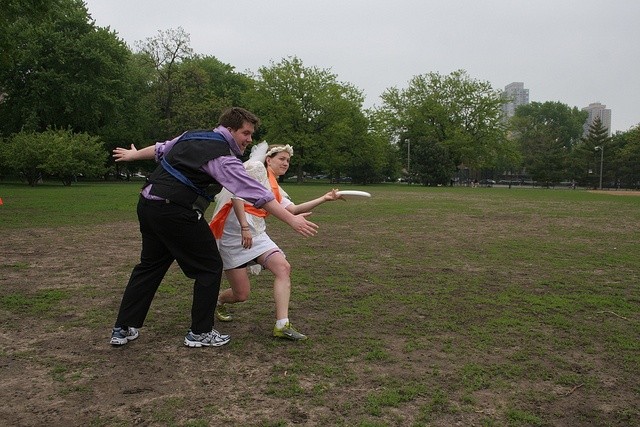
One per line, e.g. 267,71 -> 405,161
335,189 -> 371,199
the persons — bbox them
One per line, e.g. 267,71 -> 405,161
208,140 -> 347,342
109,107 -> 320,349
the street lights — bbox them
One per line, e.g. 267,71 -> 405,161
405,138 -> 410,172
595,146 -> 605,189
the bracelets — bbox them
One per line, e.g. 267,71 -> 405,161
241,223 -> 250,231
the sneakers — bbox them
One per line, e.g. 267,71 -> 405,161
110,326 -> 140,346
183,328 -> 231,347
272,322 -> 308,340
215,304 -> 233,321
